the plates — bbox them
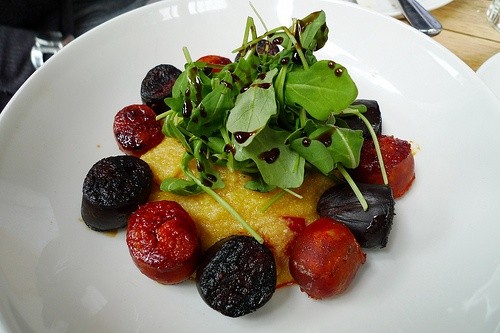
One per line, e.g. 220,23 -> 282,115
345,0 -> 454,20
476,51 -> 500,102
0,0 -> 500,333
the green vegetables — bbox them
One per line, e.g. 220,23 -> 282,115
154,9 -> 389,243
